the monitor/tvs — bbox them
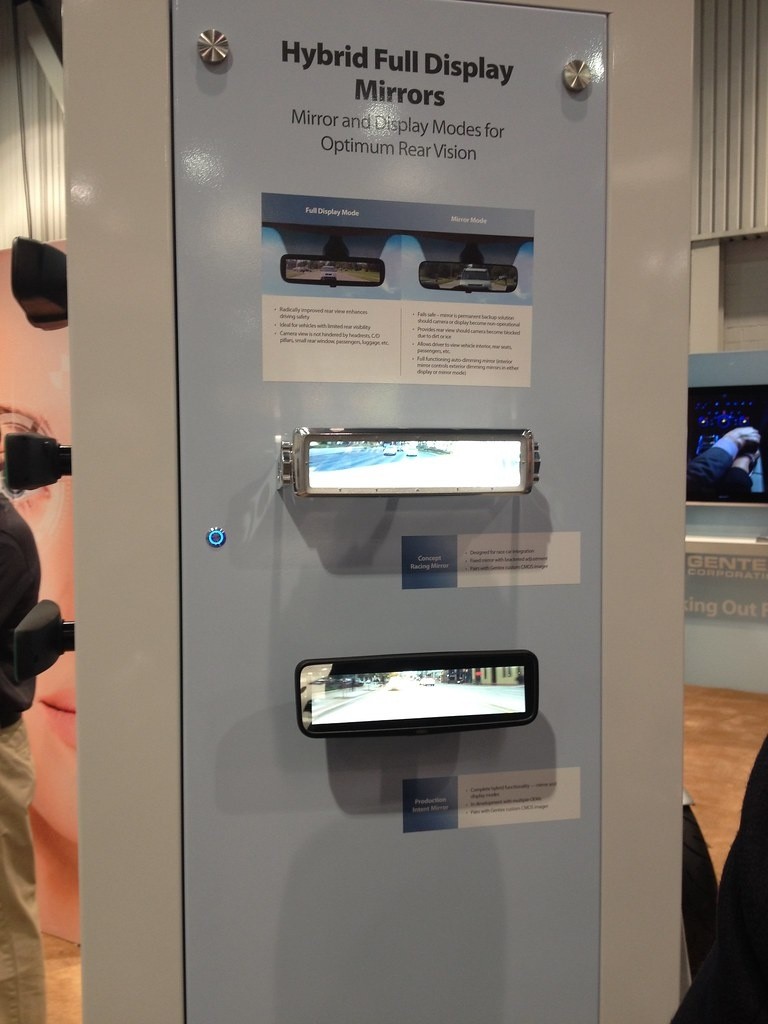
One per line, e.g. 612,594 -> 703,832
687,385 -> 768,507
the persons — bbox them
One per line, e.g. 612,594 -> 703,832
687,425 -> 762,494
